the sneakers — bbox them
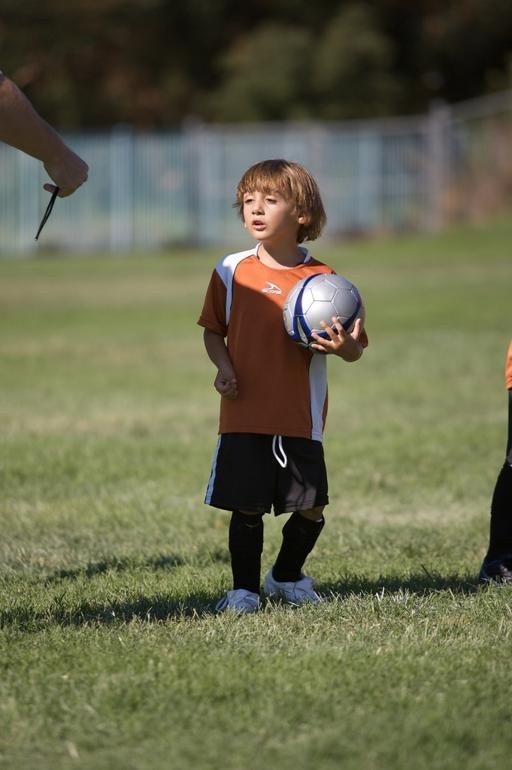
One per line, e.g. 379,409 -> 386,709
262,566 -> 323,608
216,588 -> 260,617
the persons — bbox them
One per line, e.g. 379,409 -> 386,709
194,158 -> 367,616
0,69 -> 88,198
469,334 -> 512,592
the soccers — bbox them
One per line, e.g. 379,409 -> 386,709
283,273 -> 361,352
